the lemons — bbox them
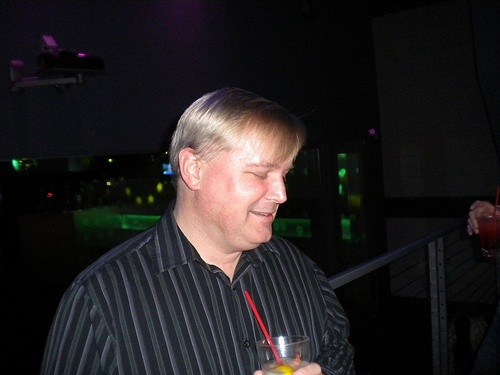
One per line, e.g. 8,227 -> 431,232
275,366 -> 295,375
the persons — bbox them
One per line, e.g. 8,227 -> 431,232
467,199 -> 500,237
39,89 -> 357,375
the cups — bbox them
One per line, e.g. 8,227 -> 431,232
256,335 -> 310,375
